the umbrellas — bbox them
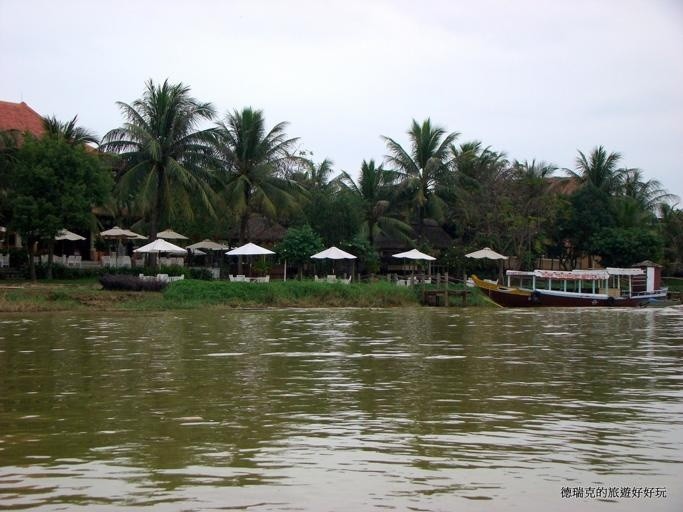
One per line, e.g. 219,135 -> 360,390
310,246 -> 357,274
56,227 -> 277,278
392,248 -> 437,288
465,247 -> 509,279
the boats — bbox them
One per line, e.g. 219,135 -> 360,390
469,259 -> 651,307
